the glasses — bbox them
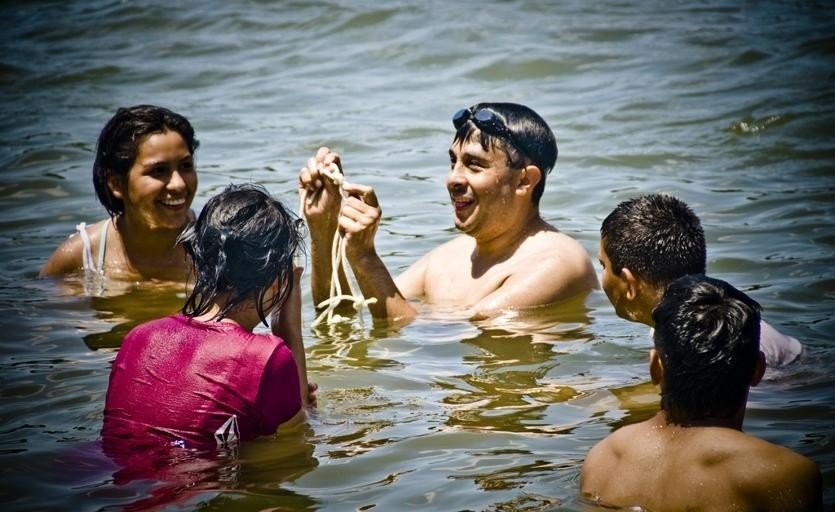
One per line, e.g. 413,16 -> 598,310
452,108 -> 527,161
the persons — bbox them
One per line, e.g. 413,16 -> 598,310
40,105 -> 207,297
54,184 -> 319,512
301,102 -> 601,330
597,192 -> 807,370
579,274 -> 823,512
